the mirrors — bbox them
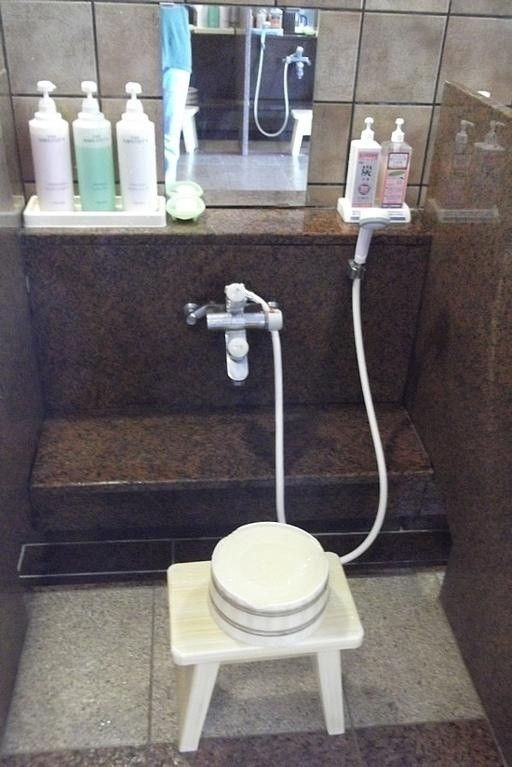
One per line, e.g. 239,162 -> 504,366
157,1 -> 319,208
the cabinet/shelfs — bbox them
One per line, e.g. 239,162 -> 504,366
187,28 -> 314,100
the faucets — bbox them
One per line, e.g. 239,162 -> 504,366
223,329 -> 254,388
296,61 -> 304,79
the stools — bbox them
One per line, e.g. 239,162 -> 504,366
160,550 -> 366,753
288,109 -> 313,160
178,105 -> 200,154
